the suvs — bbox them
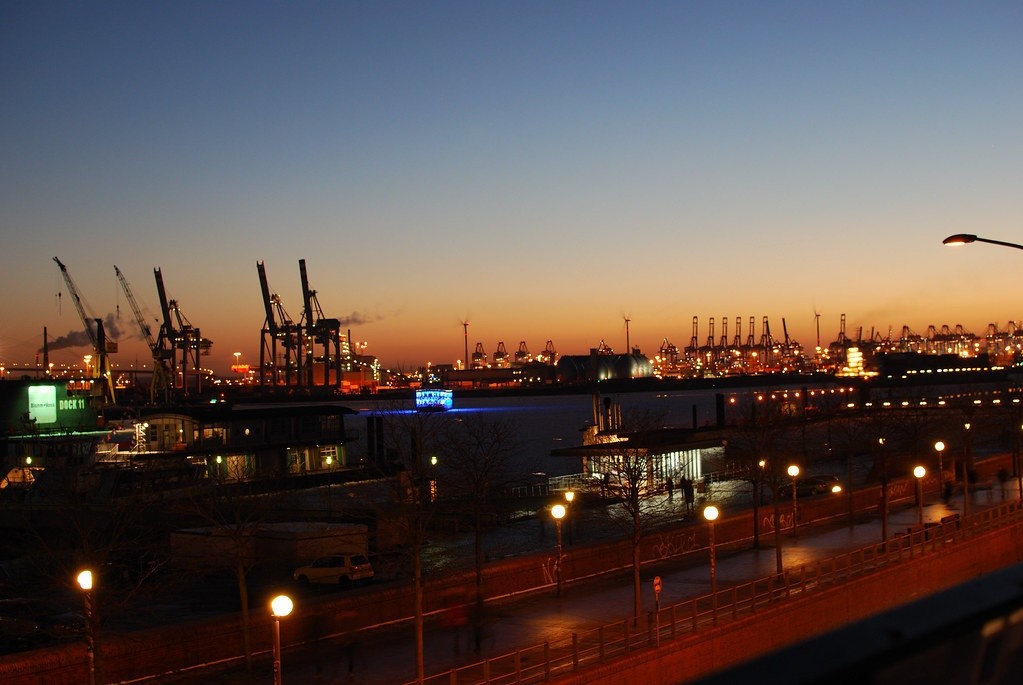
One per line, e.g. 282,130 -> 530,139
292,554 -> 374,589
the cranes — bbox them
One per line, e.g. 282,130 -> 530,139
48,257 -> 341,400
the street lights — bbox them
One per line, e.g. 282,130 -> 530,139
704,505 -> 718,592
935,442 -> 945,497
787,465 -> 800,543
913,466 -> 926,529
269,595 -> 293,685
550,504 -> 566,599
76,569 -> 94,685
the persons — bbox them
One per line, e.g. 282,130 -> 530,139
968,465 -> 1011,500
449,593 -> 483,657
680,476 -> 694,510
666,477 -> 673,497
299,610 -> 361,677
